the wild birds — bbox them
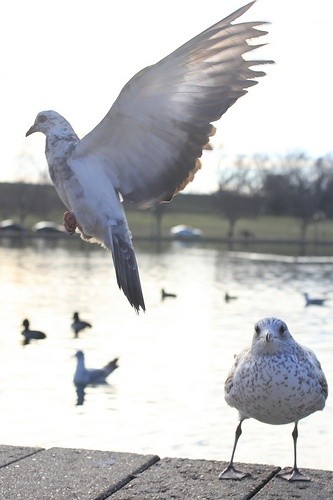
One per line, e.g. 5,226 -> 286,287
218,318 -> 329,482
304,293 -> 326,305
161,289 -> 176,297
24,0 -> 275,314
22,319 -> 47,339
71,312 -> 91,338
225,293 -> 237,301
71,351 -> 119,384
